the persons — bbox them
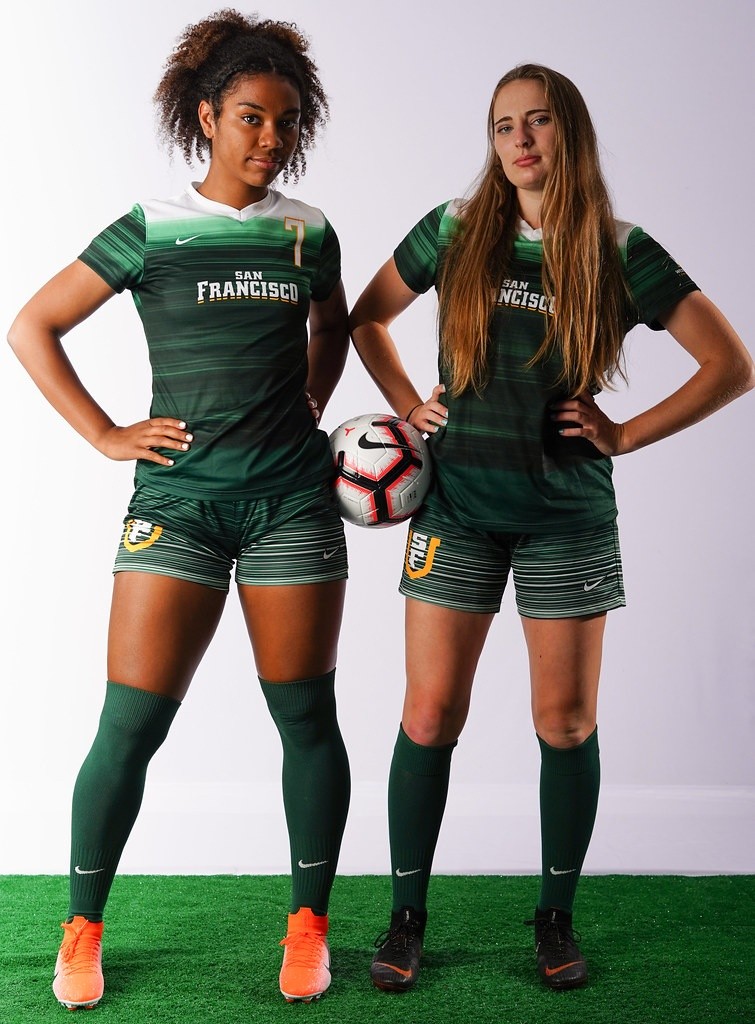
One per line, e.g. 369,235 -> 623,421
3,7 -> 352,1010
351,62 -> 754,988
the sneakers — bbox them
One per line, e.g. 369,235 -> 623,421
278,907 -> 333,1002
370,905 -> 428,989
523,907 -> 586,987
50,913 -> 106,1009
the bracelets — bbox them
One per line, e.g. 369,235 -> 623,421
405,403 -> 424,422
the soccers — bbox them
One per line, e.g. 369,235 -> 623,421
325,413 -> 432,528
